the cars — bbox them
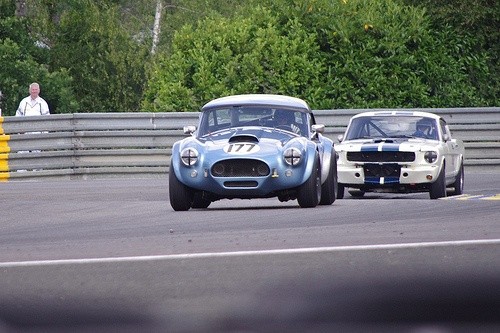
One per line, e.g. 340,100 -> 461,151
167,92 -> 341,206
331,112 -> 466,198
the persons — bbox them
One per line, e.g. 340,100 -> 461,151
271,109 -> 301,136
413,119 -> 435,140
12,82 -> 52,173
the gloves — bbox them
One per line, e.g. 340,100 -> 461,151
291,123 -> 301,134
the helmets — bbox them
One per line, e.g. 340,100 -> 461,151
415,118 -> 435,138
272,108 -> 295,132
